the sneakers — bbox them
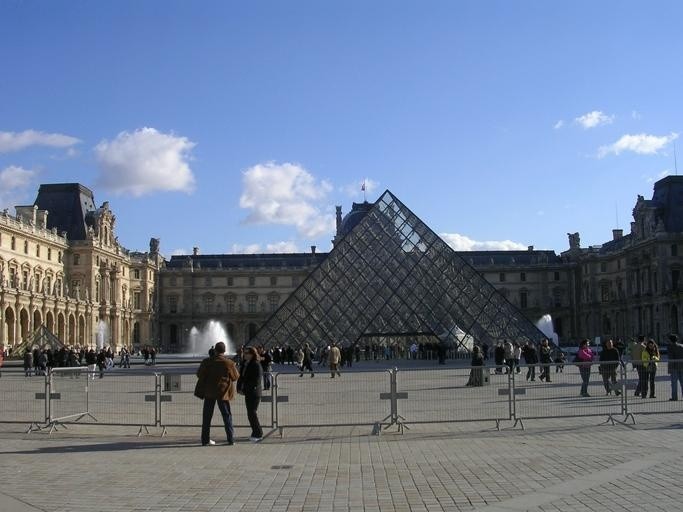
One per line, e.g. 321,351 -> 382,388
580,392 -> 590,397
204,439 -> 215,445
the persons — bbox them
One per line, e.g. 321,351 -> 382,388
237,346 -> 264,442
195,342 -> 240,445
0,342 -> 166,379
472,338 -> 564,382
233,338 -> 457,368
299,343 -> 314,377
577,339 -> 594,397
599,337 -> 682,401
208,346 -> 216,357
328,342 -> 341,378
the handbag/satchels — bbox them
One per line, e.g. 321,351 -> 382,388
572,356 -> 584,367
